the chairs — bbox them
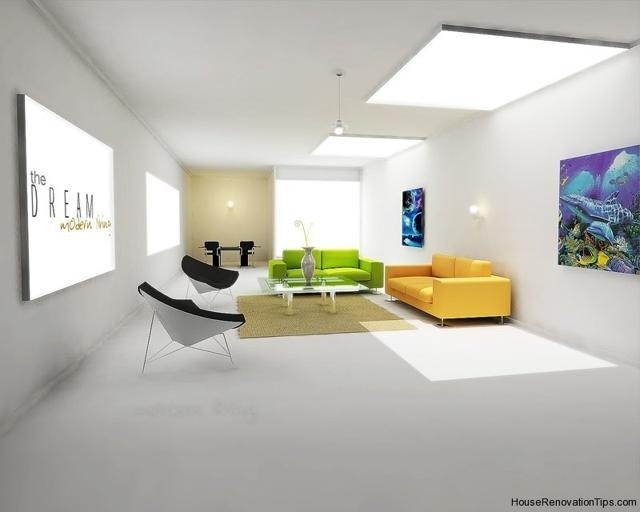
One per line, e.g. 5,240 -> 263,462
138,282 -> 246,375
238,241 -> 255,268
182,255 -> 239,306
203,242 -> 221,267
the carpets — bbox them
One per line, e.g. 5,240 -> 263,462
237,294 -> 417,338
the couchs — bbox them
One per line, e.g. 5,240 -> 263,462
386,264 -> 511,328
268,257 -> 384,295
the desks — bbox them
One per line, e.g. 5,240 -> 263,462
198,246 -> 261,266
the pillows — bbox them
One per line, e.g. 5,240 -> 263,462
454,257 -> 490,277
432,253 -> 456,277
322,248 -> 359,269
282,249 -> 321,269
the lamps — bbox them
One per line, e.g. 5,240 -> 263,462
334,74 -> 345,135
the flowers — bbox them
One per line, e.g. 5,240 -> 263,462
294,220 -> 315,246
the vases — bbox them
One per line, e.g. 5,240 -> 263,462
301,247 -> 316,286
